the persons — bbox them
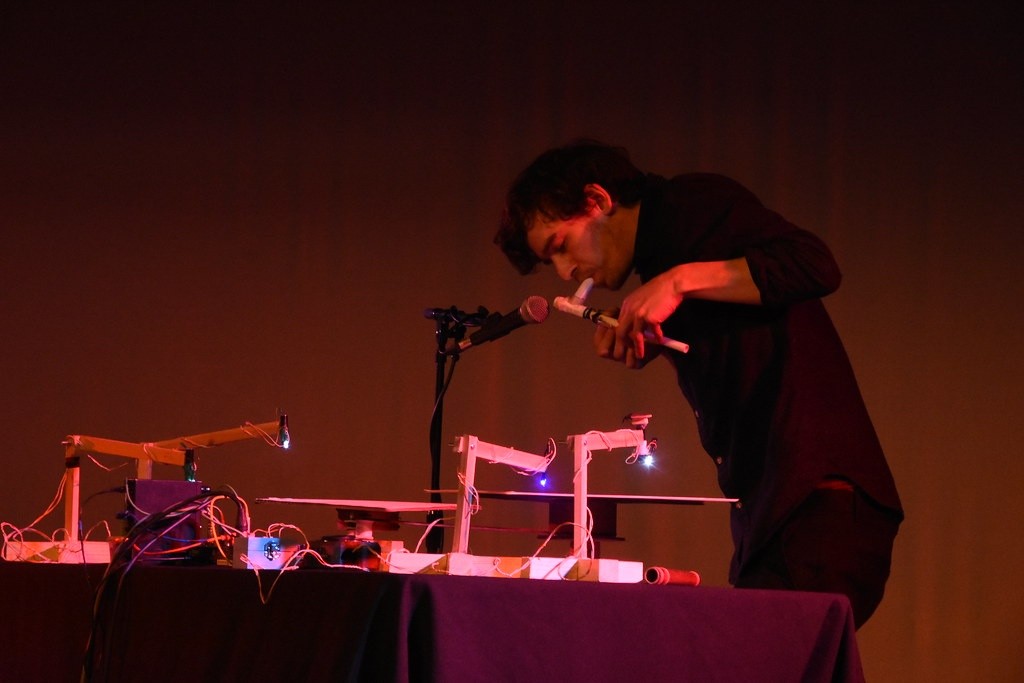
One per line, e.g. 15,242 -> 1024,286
495,142 -> 905,633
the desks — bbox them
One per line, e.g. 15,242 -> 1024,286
0,560 -> 869,683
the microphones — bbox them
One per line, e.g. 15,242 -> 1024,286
445,296 -> 551,355
